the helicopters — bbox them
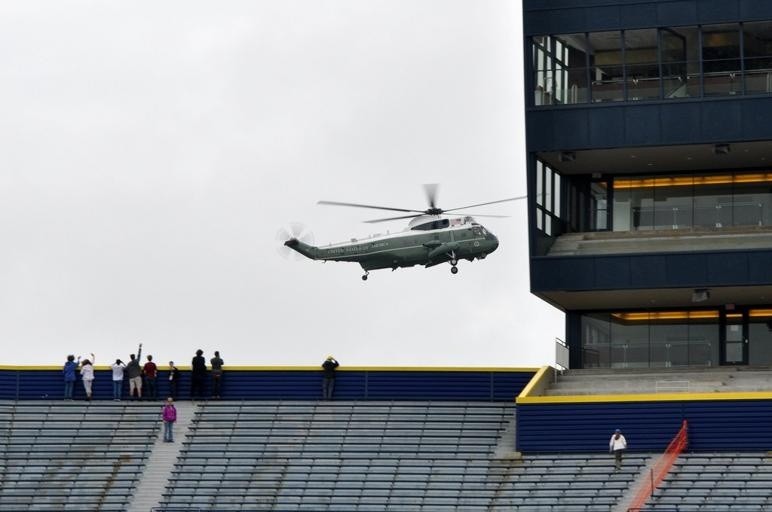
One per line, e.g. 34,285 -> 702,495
282,183 -> 550,282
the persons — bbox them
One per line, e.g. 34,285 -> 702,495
63,355 -> 81,399
166,361 -> 182,395
144,355 -> 158,400
209,350 -> 226,399
320,357 -> 338,398
163,397 -> 177,443
190,349 -> 207,400
126,343 -> 144,399
81,353 -> 95,400
607,427 -> 629,471
111,359 -> 128,401
